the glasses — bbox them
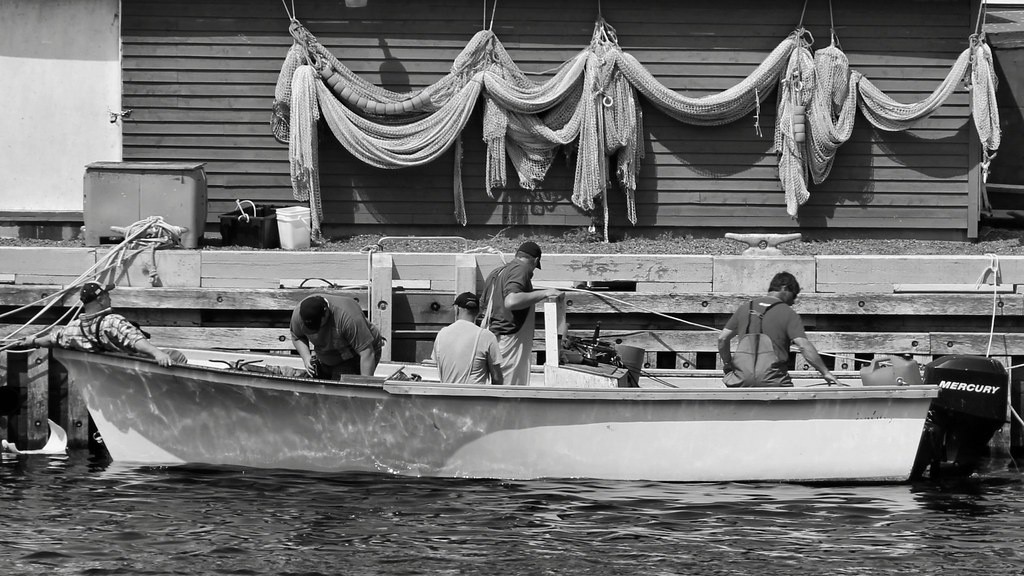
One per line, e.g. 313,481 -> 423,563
787,285 -> 797,299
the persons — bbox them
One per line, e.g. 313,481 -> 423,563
289,294 -> 387,381
24,281 -> 188,367
430,242 -> 561,384
718,270 -> 843,387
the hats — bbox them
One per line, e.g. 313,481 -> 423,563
518,241 -> 542,270
300,297 -> 323,331
80,283 -> 115,304
452,292 -> 479,310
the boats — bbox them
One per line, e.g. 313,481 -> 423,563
48,344 -> 945,484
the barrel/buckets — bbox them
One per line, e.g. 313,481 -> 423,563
276,206 -> 315,249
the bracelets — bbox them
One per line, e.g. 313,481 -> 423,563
32,338 -> 37,344
